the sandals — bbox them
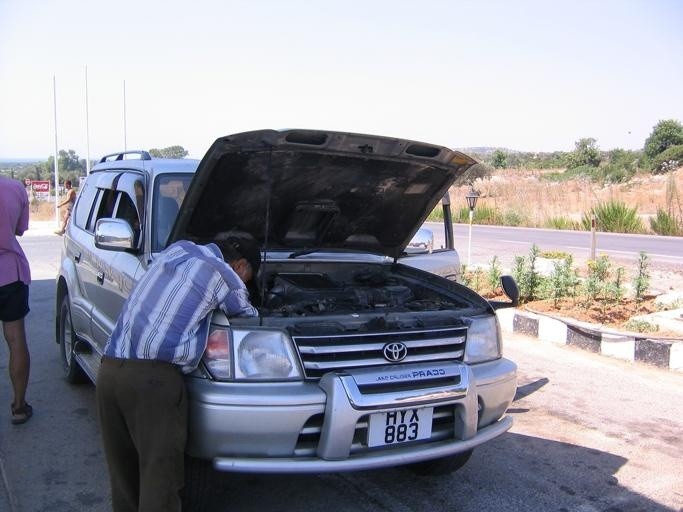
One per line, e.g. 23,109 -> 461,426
9,401 -> 33,424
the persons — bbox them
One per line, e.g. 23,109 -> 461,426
96,229 -> 259,512
0,175 -> 33,425
54,180 -> 77,236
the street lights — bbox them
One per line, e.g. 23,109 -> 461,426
462,185 -> 481,268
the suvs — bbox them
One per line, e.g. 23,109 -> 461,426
50,129 -> 515,493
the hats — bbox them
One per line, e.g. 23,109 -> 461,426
213,230 -> 260,267
